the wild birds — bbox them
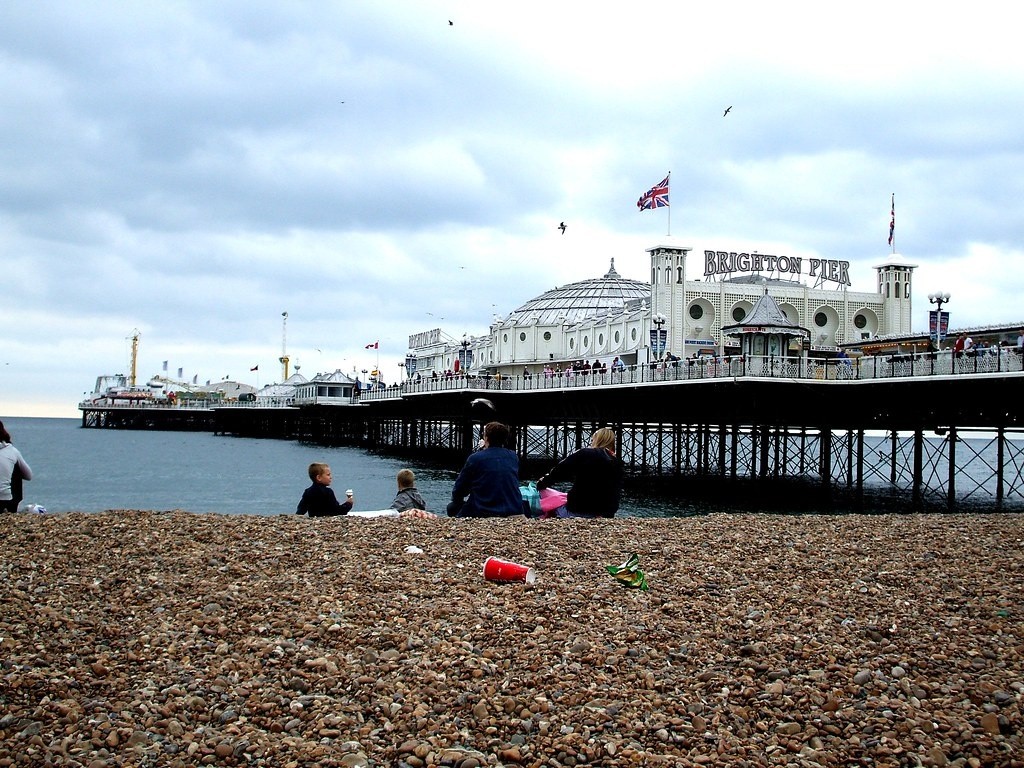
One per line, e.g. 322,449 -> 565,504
315,348 -> 322,354
470,397 -> 497,411
723,105 -> 732,116
558,222 -> 568,234
250,365 -> 259,371
425,312 -> 434,316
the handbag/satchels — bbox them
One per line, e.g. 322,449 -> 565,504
519,482 -> 546,515
400,508 -> 438,520
540,492 -> 568,520
521,499 -> 532,518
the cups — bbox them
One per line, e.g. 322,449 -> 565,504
482,555 -> 535,584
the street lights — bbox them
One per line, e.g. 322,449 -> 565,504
928,292 -> 950,351
405,348 -> 417,377
461,338 -> 472,374
653,312 -> 667,361
362,369 -> 368,390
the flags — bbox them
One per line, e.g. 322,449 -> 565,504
250,364 -> 259,371
365,341 -> 379,350
636,172 -> 670,212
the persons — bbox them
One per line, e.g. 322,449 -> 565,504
446,422 -> 525,519
0,421 -> 33,513
944,327 -> 1024,373
386,468 -> 426,512
536,427 -> 626,518
294,462 -> 354,517
835,347 -> 852,379
385,350 -> 724,390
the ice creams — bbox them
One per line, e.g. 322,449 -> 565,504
345,489 -> 354,498
